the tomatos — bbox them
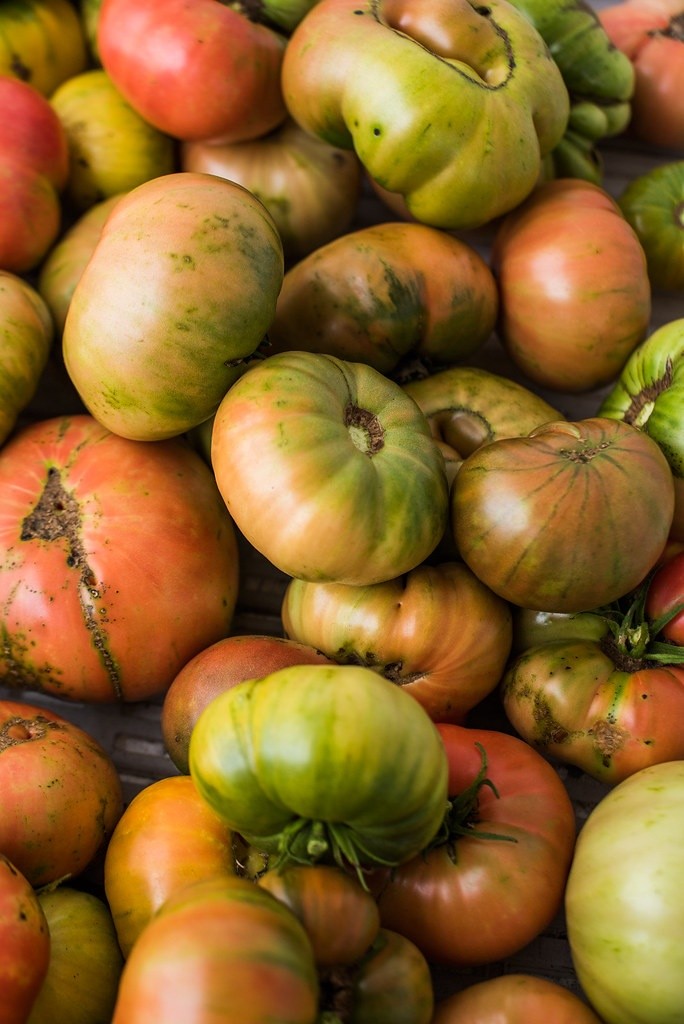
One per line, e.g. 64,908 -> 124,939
0,0 -> 684,1024
